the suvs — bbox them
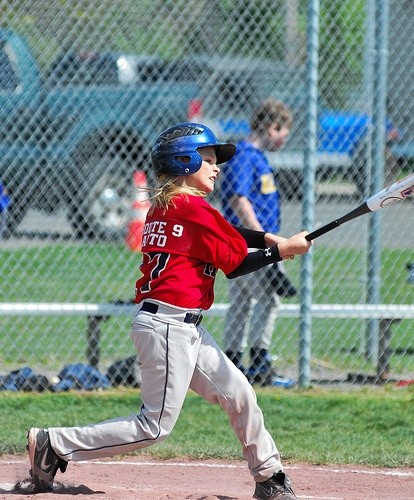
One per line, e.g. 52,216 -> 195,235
1,26 -> 414,240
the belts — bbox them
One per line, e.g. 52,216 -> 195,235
141,302 -> 205,326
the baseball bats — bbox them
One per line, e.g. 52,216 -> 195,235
304,175 -> 414,241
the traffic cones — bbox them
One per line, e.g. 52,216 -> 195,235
120,170 -> 151,251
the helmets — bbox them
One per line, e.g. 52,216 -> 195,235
151,122 -> 236,176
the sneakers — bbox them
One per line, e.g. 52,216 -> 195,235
245,365 -> 295,388
253,470 -> 299,500
26,428 -> 67,492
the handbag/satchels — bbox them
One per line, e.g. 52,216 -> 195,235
106,355 -> 142,390
0,367 -> 56,392
52,364 -> 112,391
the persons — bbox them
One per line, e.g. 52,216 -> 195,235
26,124 -> 313,500
224,95 -> 297,388
